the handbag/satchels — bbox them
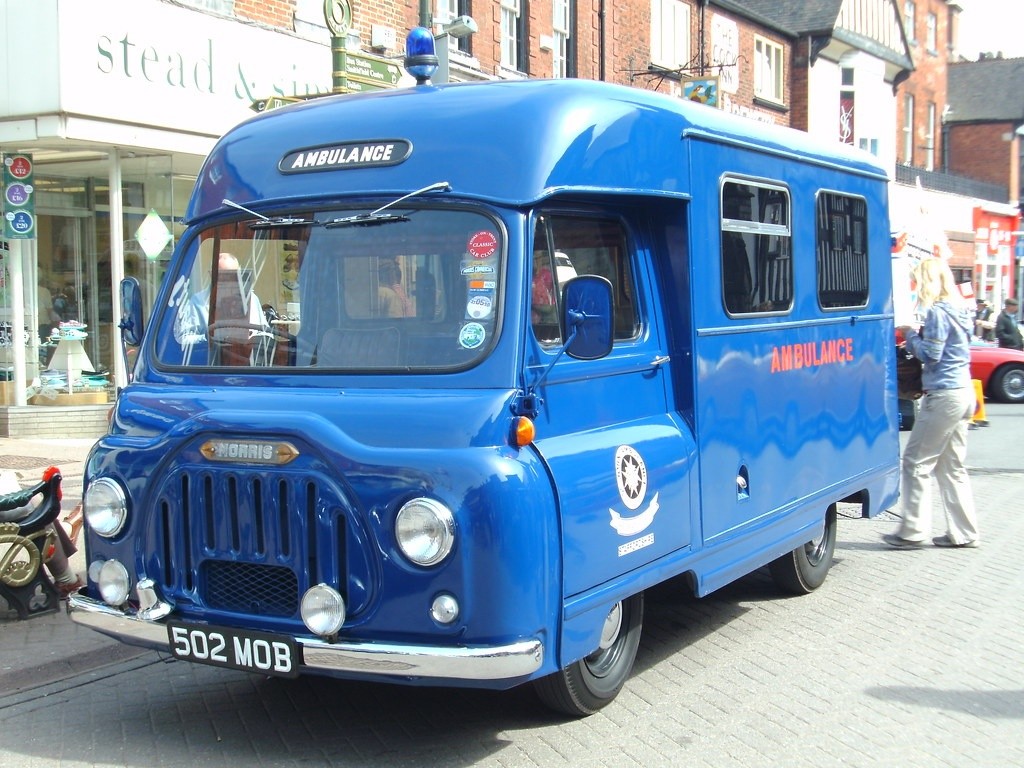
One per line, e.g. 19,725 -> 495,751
895,344 -> 923,400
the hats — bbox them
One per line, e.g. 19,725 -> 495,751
975,299 -> 988,306
1004,298 -> 1019,305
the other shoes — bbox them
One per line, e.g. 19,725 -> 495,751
932,535 -> 979,547
881,533 -> 924,550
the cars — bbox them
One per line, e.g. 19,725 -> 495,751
968,337 -> 1024,403
895,327 -> 923,400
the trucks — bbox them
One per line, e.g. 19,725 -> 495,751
66,27 -> 909,718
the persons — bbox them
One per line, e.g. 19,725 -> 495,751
0,469 -> 86,601
38,265 -> 61,372
379,259 -> 413,317
972,299 -> 995,344
123,253 -> 159,374
55,217 -> 85,270
177,253 -> 275,345
882,258 -> 980,550
995,298 -> 1024,351
